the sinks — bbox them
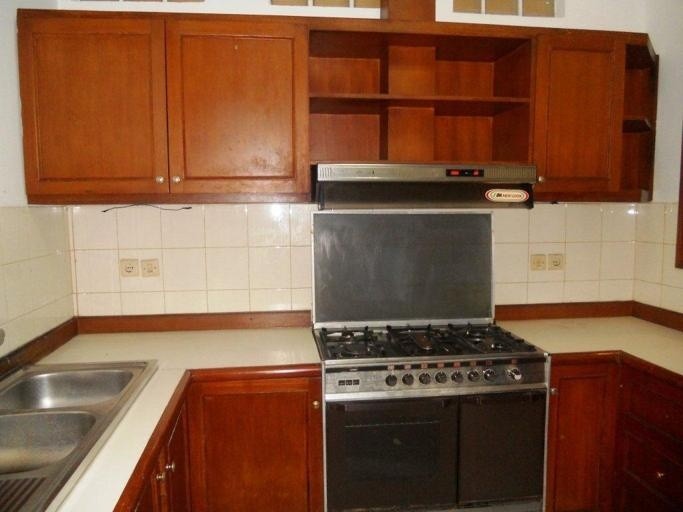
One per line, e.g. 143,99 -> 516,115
0,411 -> 96,475
0,359 -> 158,410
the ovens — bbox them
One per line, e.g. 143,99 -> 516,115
324,363 -> 548,511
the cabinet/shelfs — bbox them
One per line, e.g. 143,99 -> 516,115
551,363 -> 683,510
537,29 -> 657,191
125,370 -> 323,511
17,10 -> 309,203
310,22 -> 533,168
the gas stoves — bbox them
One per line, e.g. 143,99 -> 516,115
313,320 -> 545,363
